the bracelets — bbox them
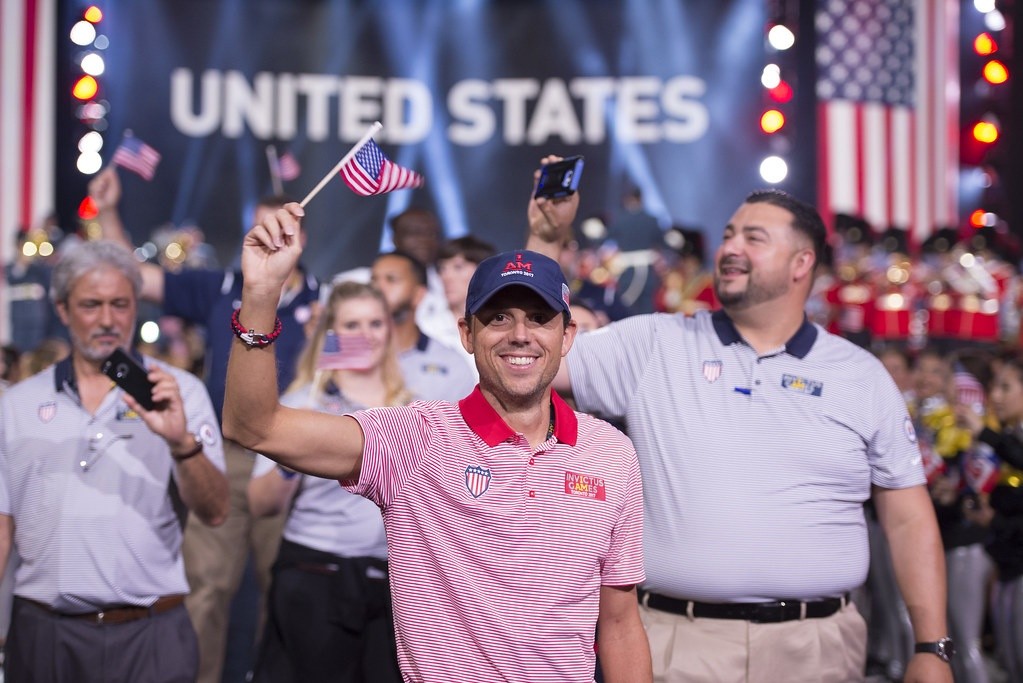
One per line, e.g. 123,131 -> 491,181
230,307 -> 287,350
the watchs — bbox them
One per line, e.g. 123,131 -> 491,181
911,634 -> 959,662
169,436 -> 205,464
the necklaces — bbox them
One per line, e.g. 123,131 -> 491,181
544,399 -> 555,441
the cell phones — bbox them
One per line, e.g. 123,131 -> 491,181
100,346 -> 161,412
534,155 -> 584,198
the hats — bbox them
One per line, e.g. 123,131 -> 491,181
655,228 -> 705,262
465,249 -> 571,318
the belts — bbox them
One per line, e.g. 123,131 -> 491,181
28,594 -> 184,624
282,556 -> 388,581
638,588 -> 850,623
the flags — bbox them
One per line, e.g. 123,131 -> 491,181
817,1 -> 959,255
109,133 -> 161,181
337,121 -> 423,198
264,144 -> 303,183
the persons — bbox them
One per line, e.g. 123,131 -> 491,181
876,341 -> 1023,683
248,281 -> 412,683
525,154 -> 955,683
0,242 -> 230,683
89,128 -> 324,683
221,202 -> 652,683
319,202 -> 501,400
